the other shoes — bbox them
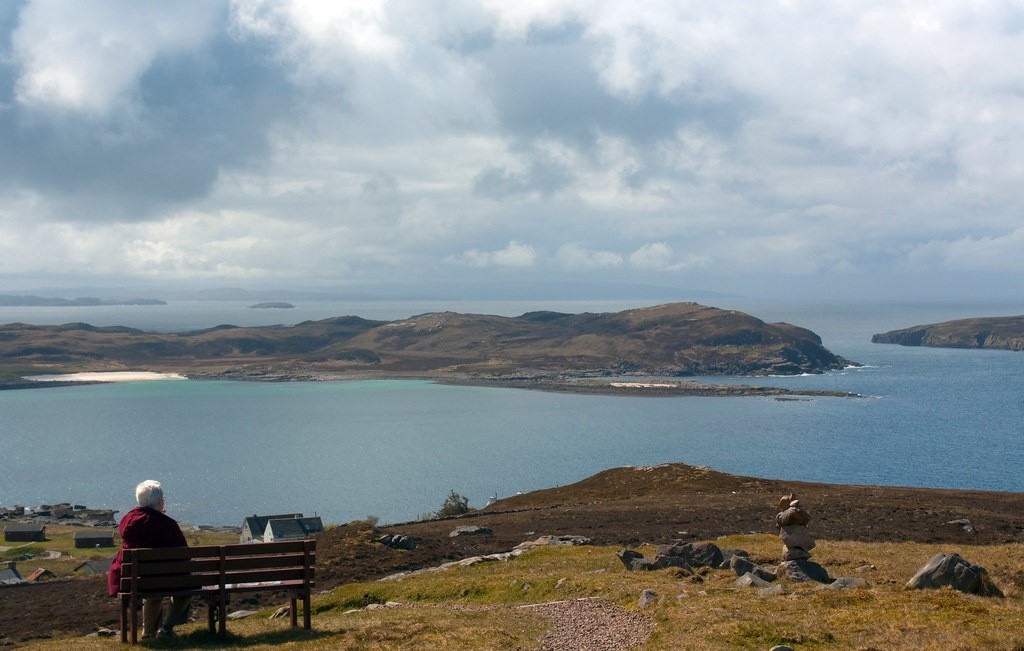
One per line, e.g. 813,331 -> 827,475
141,631 -> 159,642
156,628 -> 179,640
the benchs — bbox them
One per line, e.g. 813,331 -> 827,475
117,540 -> 316,646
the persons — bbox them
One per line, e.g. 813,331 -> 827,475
107,479 -> 190,644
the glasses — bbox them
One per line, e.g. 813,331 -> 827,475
160,497 -> 166,501
